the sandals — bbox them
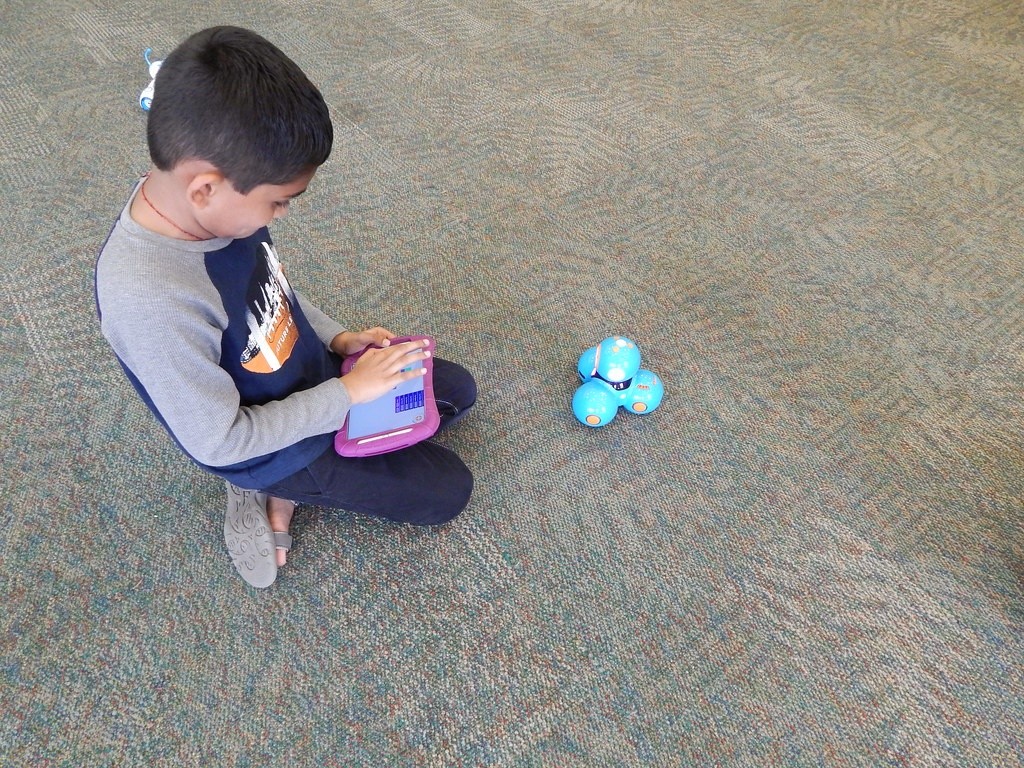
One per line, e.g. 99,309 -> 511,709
224,475 -> 299,588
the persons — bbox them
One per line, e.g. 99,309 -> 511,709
94,27 -> 479,587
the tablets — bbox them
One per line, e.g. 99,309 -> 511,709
334,335 -> 440,457
